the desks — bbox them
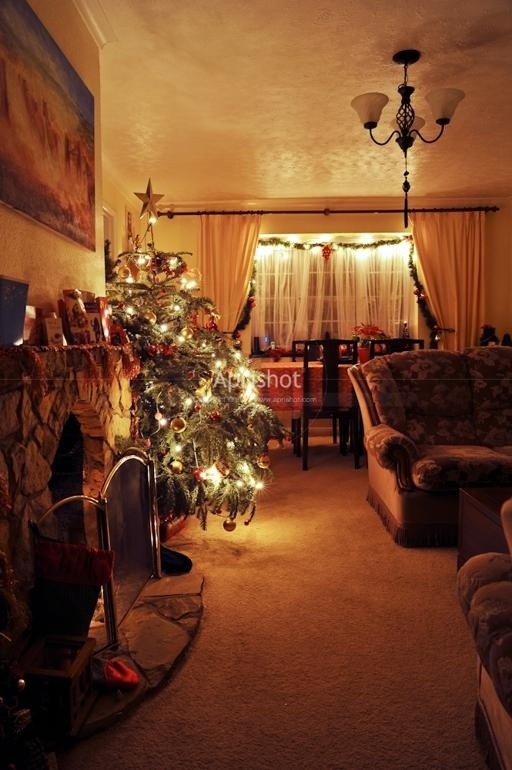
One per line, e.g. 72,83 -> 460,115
249,358 -> 358,410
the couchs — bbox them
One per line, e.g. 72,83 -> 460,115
347,346 -> 512,549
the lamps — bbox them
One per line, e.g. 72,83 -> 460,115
350,50 -> 465,230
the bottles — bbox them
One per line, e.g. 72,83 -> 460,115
400,320 -> 410,350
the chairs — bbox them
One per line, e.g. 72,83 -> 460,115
292,339 -> 424,469
